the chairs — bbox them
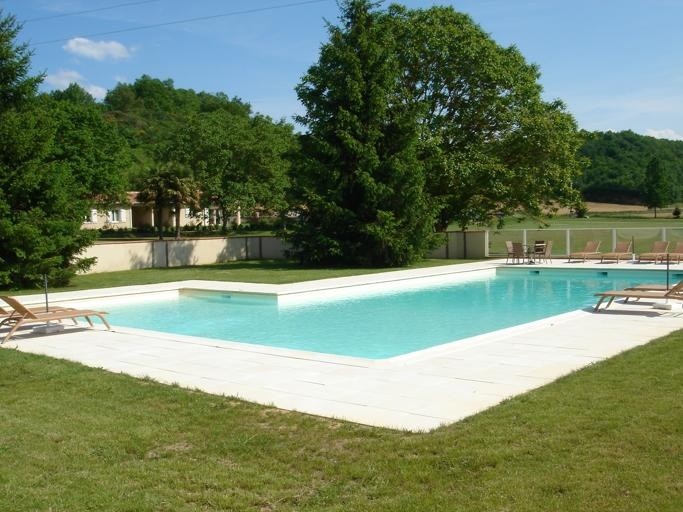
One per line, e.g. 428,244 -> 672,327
593,279 -> 683,315
0,293 -> 115,346
505,238 -> 683,267
